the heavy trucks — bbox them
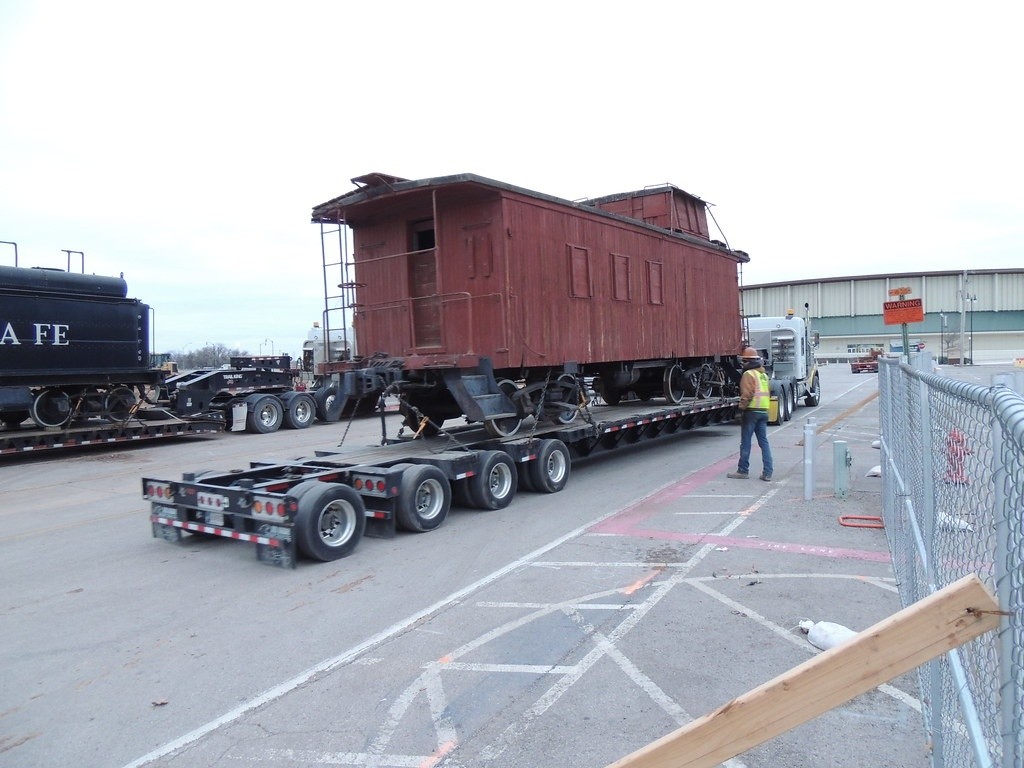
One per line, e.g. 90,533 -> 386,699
138,170 -> 823,572
0,240 -> 378,457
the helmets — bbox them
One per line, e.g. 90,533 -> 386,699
739,347 -> 760,358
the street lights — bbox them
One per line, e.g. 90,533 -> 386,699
966,294 -> 978,363
940,309 -> 945,364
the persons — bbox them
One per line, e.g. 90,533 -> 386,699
728,348 -> 773,481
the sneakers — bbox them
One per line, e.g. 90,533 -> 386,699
759,474 -> 772,481
727,471 -> 749,479
